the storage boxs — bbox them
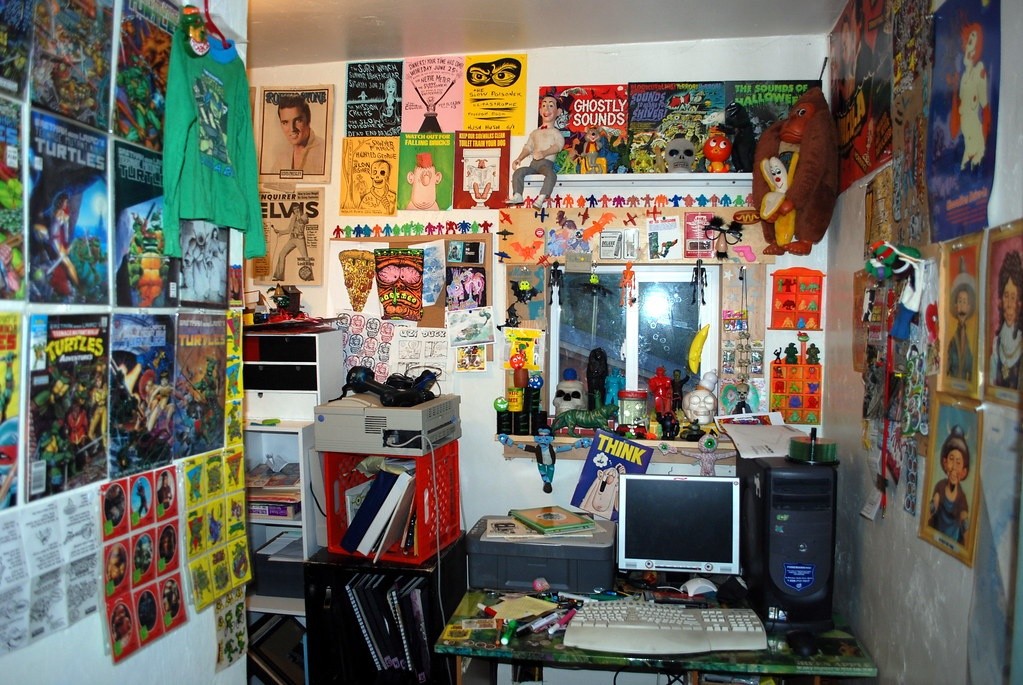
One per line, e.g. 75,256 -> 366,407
467,514 -> 617,593
323,438 -> 461,566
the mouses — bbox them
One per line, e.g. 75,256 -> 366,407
785,629 -> 819,656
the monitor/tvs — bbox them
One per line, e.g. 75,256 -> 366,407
619,474 -> 740,587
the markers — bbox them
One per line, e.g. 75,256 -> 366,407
557,591 -> 599,604
477,602 -> 497,617
514,613 -> 558,637
548,608 -> 577,634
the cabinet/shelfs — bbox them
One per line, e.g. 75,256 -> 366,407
300,528 -> 465,685
245,558 -> 301,685
241,325 -> 343,558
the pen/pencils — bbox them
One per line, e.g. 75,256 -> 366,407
495,619 -> 503,645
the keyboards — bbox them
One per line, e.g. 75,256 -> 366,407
563,602 -> 767,656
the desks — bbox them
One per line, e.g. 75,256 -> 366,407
434,588 -> 877,685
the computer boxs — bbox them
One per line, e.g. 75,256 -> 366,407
737,453 -> 836,632
